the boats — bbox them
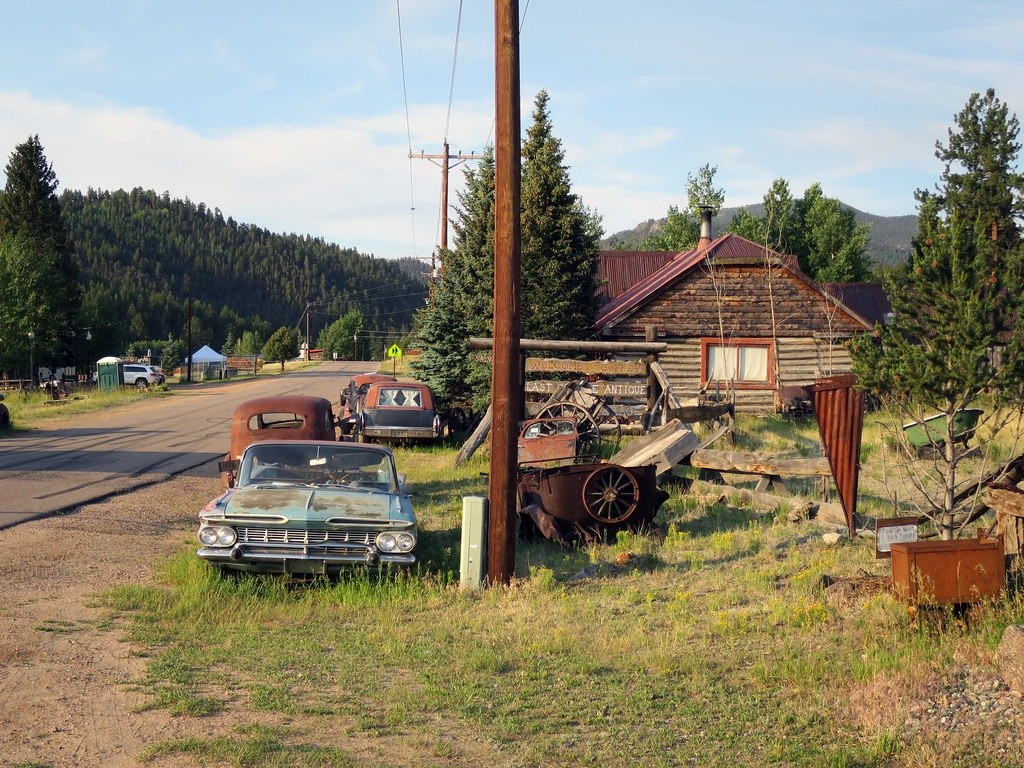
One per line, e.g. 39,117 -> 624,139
902,406 -> 985,452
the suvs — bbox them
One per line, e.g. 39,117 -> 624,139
92,363 -> 165,389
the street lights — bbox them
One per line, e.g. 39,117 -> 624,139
27,327 -> 35,390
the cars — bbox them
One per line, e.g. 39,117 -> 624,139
340,373 -> 399,435
218,395 -> 348,502
196,440 -> 418,586
358,380 -> 441,446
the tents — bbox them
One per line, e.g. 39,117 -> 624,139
185,345 -> 227,381
